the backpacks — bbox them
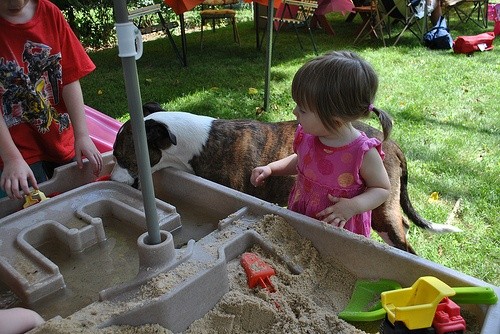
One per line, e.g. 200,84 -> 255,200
425,16 -> 452,49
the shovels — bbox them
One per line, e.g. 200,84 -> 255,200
240,253 -> 286,312
338,280 -> 498,321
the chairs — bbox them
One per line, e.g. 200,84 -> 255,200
127,0 -> 491,66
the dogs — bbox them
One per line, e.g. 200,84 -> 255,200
106,97 -> 465,257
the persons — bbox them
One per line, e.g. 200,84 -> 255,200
0,0 -> 103,200
250,52 -> 391,238
0,307 -> 46,334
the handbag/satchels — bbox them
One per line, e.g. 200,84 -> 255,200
454,31 -> 495,54
